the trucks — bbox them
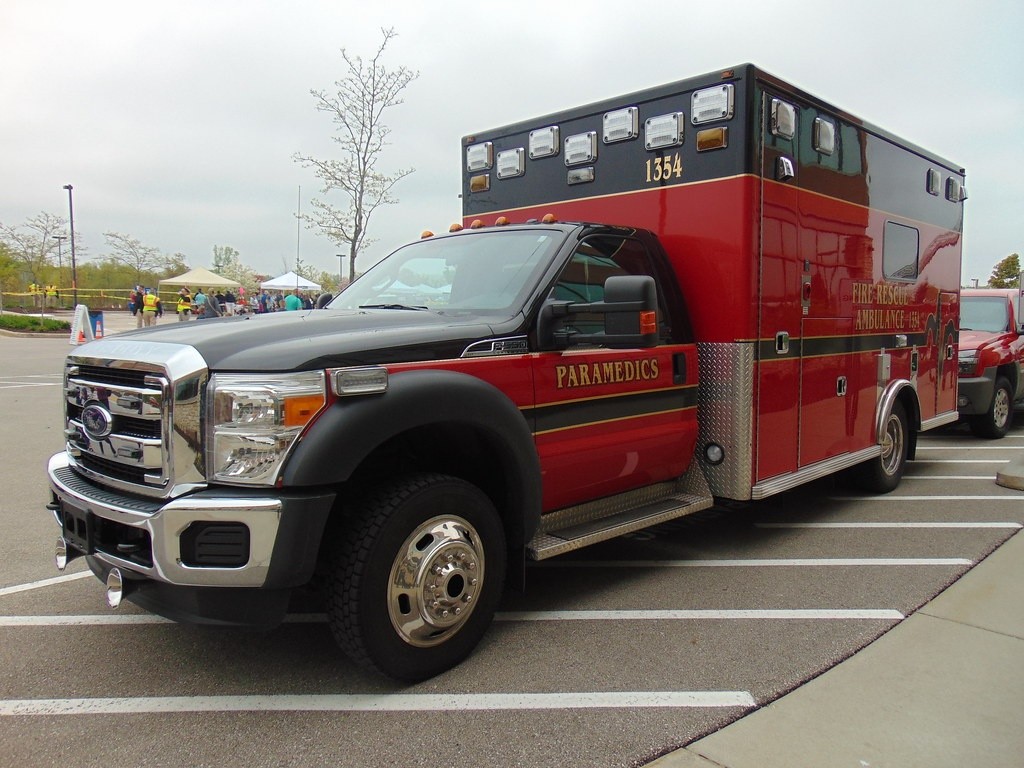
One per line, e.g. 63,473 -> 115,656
47,62 -> 967,686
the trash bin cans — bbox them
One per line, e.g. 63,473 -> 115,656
87,311 -> 104,338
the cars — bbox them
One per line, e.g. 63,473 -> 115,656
959,287 -> 1024,441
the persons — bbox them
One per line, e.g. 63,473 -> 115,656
44,281 -> 59,308
29,282 -> 43,308
250,289 -> 333,314
130,285 -> 246,329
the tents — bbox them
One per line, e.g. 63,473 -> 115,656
260,271 -> 321,298
157,268 -> 243,307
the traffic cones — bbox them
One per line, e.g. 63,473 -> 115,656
93,321 -> 102,340
